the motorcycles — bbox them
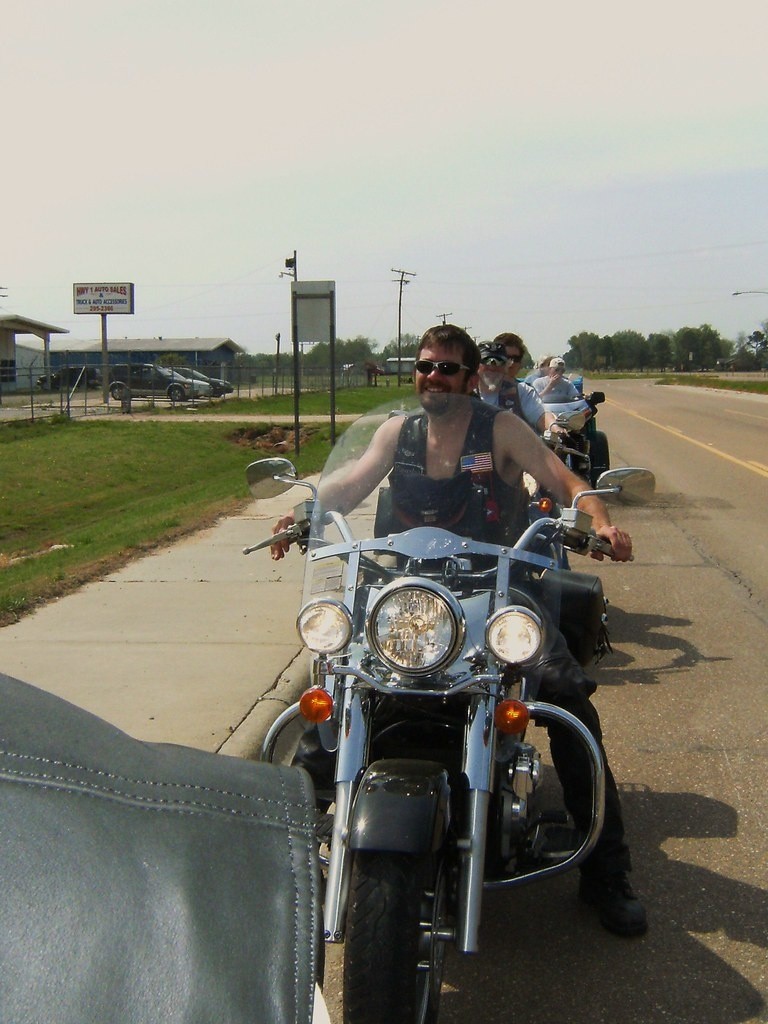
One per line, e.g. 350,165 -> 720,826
239,374 -> 656,1024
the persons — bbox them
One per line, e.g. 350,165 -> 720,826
472,331 -> 574,570
524,355 -> 592,486
272,325 -> 648,938
0,672 -> 333,1024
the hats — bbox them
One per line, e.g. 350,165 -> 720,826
549,358 -> 565,368
539,356 -> 550,368
477,340 -> 507,364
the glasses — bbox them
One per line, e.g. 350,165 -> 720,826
554,368 -> 565,373
506,354 -> 523,363
415,359 -> 471,376
481,358 -> 502,367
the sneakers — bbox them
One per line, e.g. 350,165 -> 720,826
579,868 -> 648,938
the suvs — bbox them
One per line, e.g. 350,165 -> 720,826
109,363 -> 214,401
164,366 -> 234,398
